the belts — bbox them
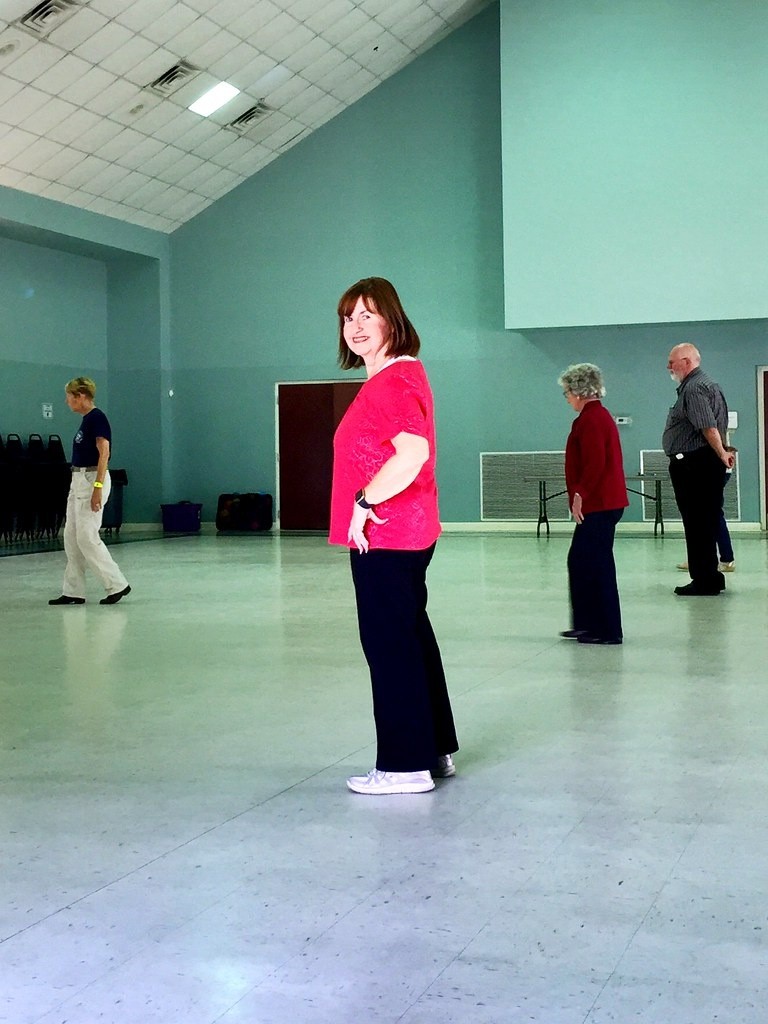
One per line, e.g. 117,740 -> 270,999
70,465 -> 108,472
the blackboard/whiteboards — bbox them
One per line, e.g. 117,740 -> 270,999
639,449 -> 744,523
479,449 -> 574,522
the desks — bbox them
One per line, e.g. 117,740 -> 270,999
524,476 -> 669,536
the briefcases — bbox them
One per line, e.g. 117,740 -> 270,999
216,493 -> 272,531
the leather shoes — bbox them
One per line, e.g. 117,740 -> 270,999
49,595 -> 85,605
100,585 -> 131,604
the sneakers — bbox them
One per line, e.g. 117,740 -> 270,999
427,753 -> 455,778
346,767 -> 435,794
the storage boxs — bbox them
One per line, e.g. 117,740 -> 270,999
160,503 -> 203,532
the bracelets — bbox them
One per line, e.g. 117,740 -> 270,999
93,482 -> 103,488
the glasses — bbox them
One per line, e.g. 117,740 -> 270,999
563,388 -> 571,398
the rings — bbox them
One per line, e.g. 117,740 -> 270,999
96,503 -> 99,506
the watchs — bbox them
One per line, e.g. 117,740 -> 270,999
354,488 -> 371,509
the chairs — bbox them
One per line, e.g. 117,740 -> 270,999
1,433 -> 73,545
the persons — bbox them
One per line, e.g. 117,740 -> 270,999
677,430 -> 735,572
48,377 -> 133,604
327,277 -> 460,795
662,342 -> 735,596
558,363 -> 630,645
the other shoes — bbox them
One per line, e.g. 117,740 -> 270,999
676,562 -> 688,569
559,628 -> 589,637
674,574 -> 725,595
716,559 -> 735,572
577,631 -> 622,644
